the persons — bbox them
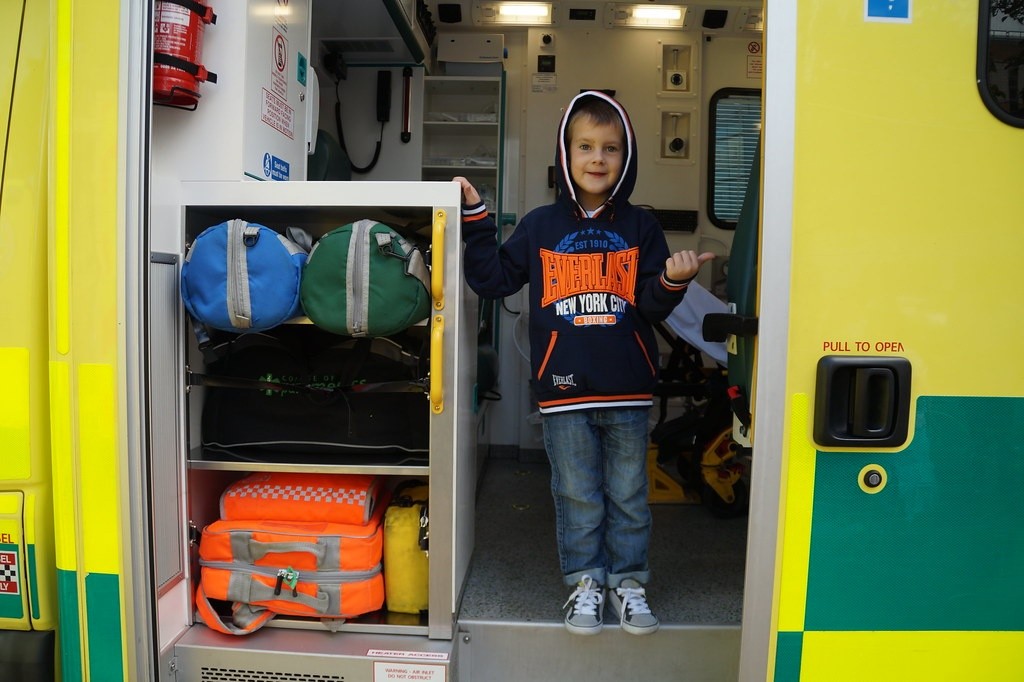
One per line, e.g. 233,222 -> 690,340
449,86 -> 715,635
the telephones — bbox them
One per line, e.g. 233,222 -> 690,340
376,70 -> 392,123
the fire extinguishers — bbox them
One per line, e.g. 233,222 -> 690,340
152,0 -> 209,106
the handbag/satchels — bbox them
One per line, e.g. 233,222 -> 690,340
379,481 -> 432,618
186,218 -> 307,335
301,221 -> 435,335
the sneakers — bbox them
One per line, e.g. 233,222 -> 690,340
606,587 -> 661,636
561,575 -> 608,634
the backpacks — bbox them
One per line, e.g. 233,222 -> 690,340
194,474 -> 386,646
190,339 -> 431,463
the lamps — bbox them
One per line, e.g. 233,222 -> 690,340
472,1 -> 561,28
605,2 -> 696,31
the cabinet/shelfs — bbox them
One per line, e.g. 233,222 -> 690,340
421,33 -> 507,413
188,180 -> 480,641
